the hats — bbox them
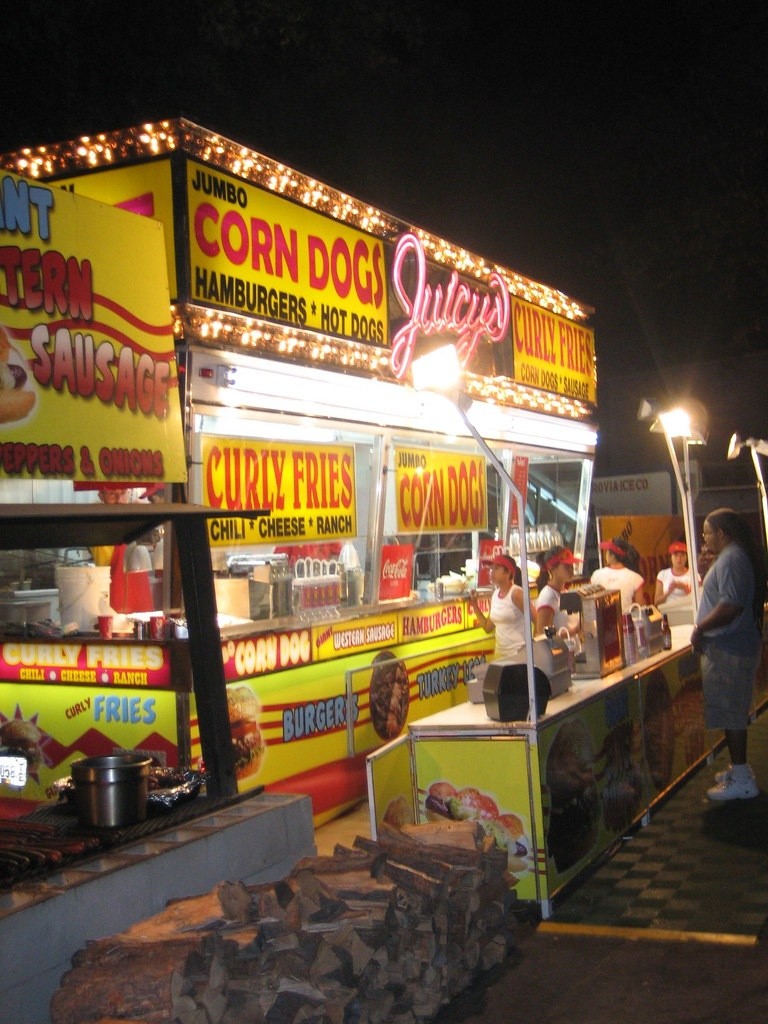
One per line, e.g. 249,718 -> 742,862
669,541 -> 687,553
139,484 -> 164,500
482,555 -> 516,575
600,538 -> 627,557
546,549 -> 580,568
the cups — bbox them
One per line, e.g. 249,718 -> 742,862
621,612 -> 639,666
435,583 -> 443,600
150,616 -> 166,639
97,615 -> 113,640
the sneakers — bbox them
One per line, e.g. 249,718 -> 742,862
706,775 -> 760,800
715,763 -> 755,783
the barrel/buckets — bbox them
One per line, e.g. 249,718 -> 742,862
55,564 -> 111,632
68,755 -> 153,828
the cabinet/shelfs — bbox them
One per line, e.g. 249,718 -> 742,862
0,502 -> 271,891
493,523 -> 564,581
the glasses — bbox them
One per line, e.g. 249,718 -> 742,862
702,530 -> 718,538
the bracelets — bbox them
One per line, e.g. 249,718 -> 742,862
695,625 -> 701,635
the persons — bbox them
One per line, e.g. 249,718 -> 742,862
469,555 -> 537,661
654,542 -> 702,626
590,537 -> 646,614
690,508 -> 764,801
534,546 -> 581,638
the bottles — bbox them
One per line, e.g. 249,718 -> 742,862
662,613 -> 672,651
495,523 -> 564,554
